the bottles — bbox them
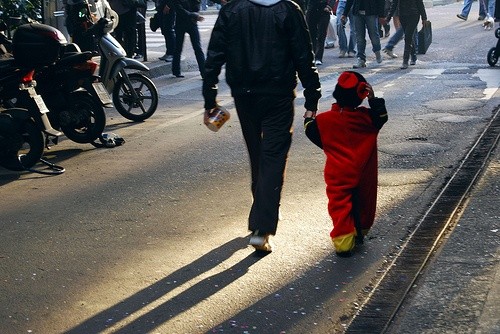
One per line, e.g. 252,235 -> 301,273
103,132 -> 119,140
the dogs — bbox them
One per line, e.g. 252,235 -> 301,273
481,12 -> 497,31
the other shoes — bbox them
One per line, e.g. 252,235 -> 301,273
457,13 -> 468,21
338,50 -> 346,58
348,51 -> 355,58
382,48 -> 398,58
375,50 -> 383,64
410,60 -> 416,65
352,57 -> 366,68
165,56 -> 173,62
315,60 -> 322,65
249,230 -> 272,253
477,15 -> 486,21
173,73 -> 185,77
400,63 -> 408,69
158,54 -> 168,60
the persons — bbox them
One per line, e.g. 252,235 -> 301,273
202,0 -> 321,253
168,0 -> 206,78
295,0 -> 427,69
304,71 -> 389,256
63,0 -> 176,62
457,0 -> 500,22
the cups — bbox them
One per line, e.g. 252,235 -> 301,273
206,106 -> 231,130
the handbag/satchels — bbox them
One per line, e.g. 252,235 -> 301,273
417,21 -> 433,54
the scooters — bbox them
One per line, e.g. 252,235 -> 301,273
0,0 -> 159,123
0,20 -> 113,173
